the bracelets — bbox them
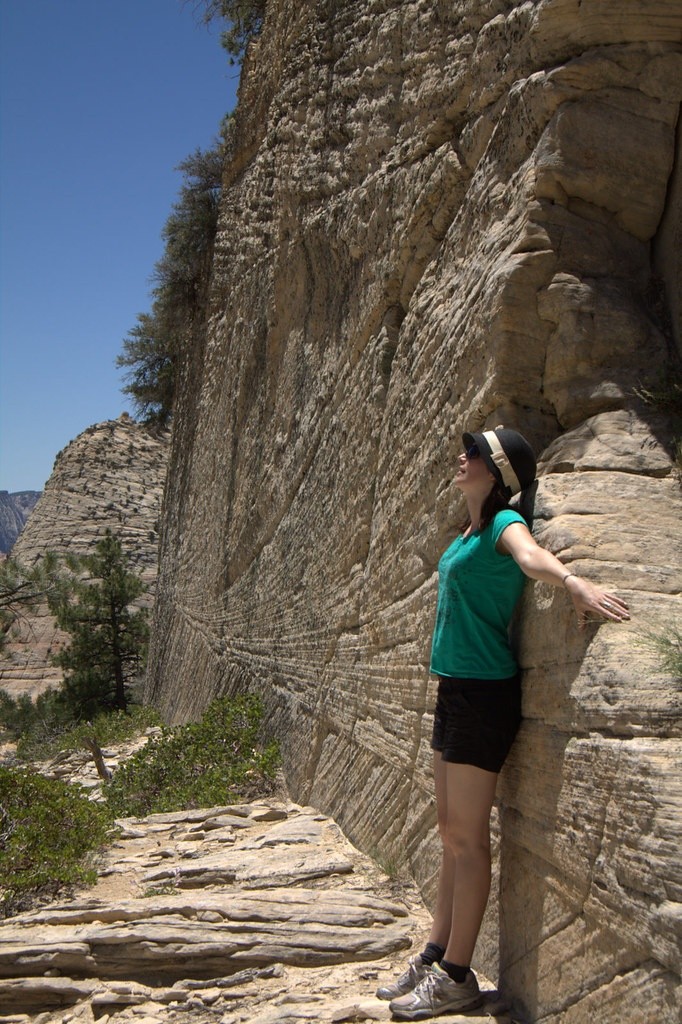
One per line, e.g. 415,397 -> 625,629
561,572 -> 579,589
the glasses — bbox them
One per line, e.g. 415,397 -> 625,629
465,445 -> 481,460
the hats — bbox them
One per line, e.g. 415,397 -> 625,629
463,429 -> 537,493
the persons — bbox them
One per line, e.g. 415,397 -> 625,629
376,427 -> 631,1021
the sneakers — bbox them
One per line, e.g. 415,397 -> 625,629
377,953 -> 482,1017
